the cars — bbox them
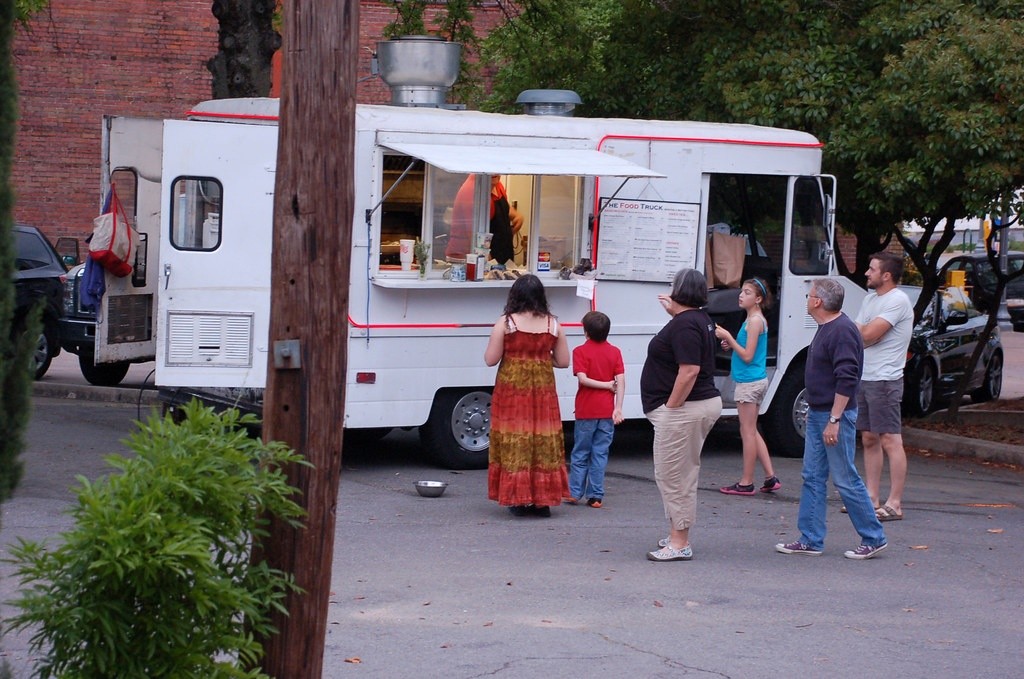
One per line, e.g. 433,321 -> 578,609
10,224 -> 67,381
938,248 -> 1024,304
898,284 -> 1005,414
51,234 -> 135,386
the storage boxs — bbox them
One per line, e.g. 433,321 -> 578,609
538,252 -> 551,271
466,253 -> 485,280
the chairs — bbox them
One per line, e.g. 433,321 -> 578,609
706,287 -> 745,314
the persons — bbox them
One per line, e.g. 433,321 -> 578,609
484,274 -> 570,518
841,254 -> 914,521
641,269 -> 723,562
715,277 -> 782,495
446,173 -> 523,264
564,312 -> 625,507
776,277 -> 888,559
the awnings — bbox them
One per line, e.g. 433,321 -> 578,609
368,143 -> 668,219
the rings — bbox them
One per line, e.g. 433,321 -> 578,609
830,438 -> 833,440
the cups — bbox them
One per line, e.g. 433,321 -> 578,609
449,263 -> 466,282
475,232 -> 493,248
474,247 -> 491,259
399,239 -> 415,270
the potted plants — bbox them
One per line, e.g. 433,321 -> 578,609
412,235 -> 432,280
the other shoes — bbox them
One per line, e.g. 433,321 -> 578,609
526,504 -> 551,517
657,538 -> 690,549
509,504 -> 529,515
648,545 -> 692,560
588,497 -> 602,507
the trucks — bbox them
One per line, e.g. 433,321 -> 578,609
93,34 -> 875,476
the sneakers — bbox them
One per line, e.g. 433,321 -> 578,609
760,477 -> 781,491
720,482 -> 755,495
775,540 -> 822,556
843,541 -> 887,559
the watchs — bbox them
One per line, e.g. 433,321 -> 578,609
829,414 -> 840,423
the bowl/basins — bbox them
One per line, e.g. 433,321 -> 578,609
413,480 -> 447,497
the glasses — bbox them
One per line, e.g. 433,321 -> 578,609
808,294 -> 824,302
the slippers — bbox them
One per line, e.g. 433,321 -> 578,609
874,505 -> 903,520
841,504 -> 879,511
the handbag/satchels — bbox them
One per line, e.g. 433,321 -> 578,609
705,229 -> 748,288
89,182 -> 139,277
